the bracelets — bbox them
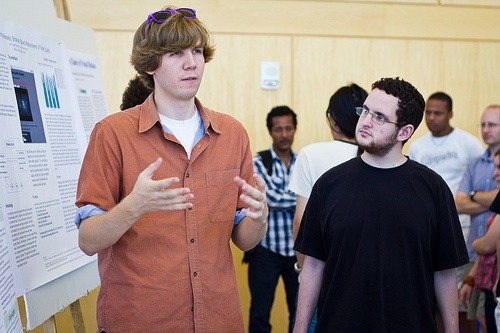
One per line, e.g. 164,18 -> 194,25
462,274 -> 474,286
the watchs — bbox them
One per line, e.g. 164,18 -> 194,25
469,190 -> 477,202
294,261 -> 301,273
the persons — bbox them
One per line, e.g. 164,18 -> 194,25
293,77 -> 469,333
75,6 -> 268,333
409,92 -> 500,333
288,82 -> 367,269
240,105 -> 301,333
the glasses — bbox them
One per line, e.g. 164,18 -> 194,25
356,105 -> 405,127
144,7 -> 197,38
